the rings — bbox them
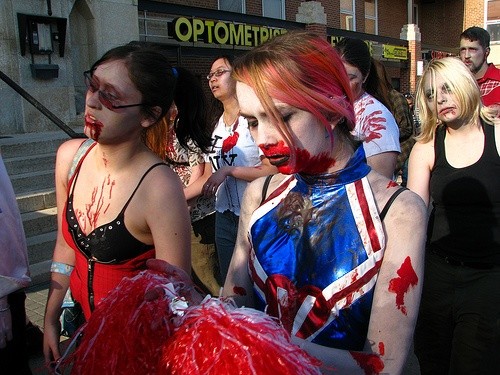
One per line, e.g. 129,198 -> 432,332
207,185 -> 209,187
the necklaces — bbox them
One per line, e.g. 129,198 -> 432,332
223,110 -> 238,126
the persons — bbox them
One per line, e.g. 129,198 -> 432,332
166,118 -> 219,299
375,62 -> 420,186
334,37 -> 401,181
183,53 -> 279,288
44,43 -> 218,374
222,32 -> 427,375
459,26 -> 500,108
407,56 -> 500,375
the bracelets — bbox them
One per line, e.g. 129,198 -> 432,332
50,262 -> 74,277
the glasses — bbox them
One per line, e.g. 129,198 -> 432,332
83,69 -> 143,112
207,69 -> 232,79
